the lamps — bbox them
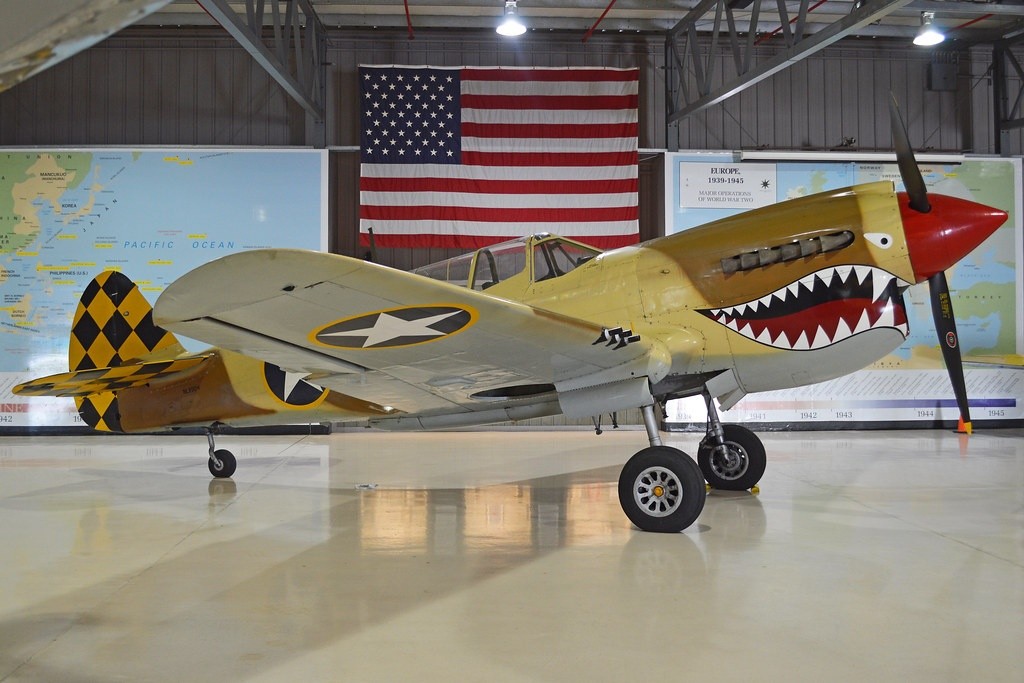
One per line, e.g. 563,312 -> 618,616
911,10 -> 945,46
496,0 -> 527,36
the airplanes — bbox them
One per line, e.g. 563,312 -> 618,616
9,94 -> 1008,534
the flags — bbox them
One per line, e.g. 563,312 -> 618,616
358,64 -> 638,254
